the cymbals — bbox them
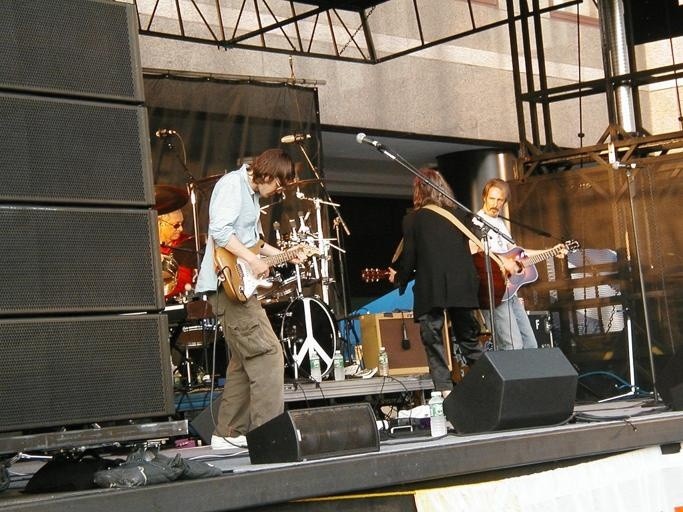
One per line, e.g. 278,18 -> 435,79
192,172 -> 225,190
152,184 -> 189,216
284,178 -> 324,193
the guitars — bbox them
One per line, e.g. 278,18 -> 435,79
360,251 -> 507,310
492,239 -> 581,303
212,240 -> 324,303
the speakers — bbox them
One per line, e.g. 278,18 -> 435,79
246,403 -> 380,464
442,346 -> 578,435
654,343 -> 683,412
0,0 -> 176,432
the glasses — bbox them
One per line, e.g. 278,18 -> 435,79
159,217 -> 184,229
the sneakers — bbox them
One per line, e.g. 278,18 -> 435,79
211,434 -> 248,450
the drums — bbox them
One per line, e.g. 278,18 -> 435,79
306,254 -> 322,286
270,262 -> 307,298
169,295 -> 227,351
262,293 -> 335,382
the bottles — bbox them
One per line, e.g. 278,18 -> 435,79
377,347 -> 389,377
429,392 -> 447,438
310,350 -> 345,382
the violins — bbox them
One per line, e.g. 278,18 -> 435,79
172,230 -> 209,270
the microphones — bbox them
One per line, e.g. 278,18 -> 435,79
356,132 -> 381,148
156,129 -> 176,137
403,324 -> 410,349
612,161 -> 644,170
280,133 -> 311,143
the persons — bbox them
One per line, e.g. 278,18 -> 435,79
157,206 -> 198,303
385,168 -> 482,399
194,148 -> 308,450
468,177 -> 568,349
